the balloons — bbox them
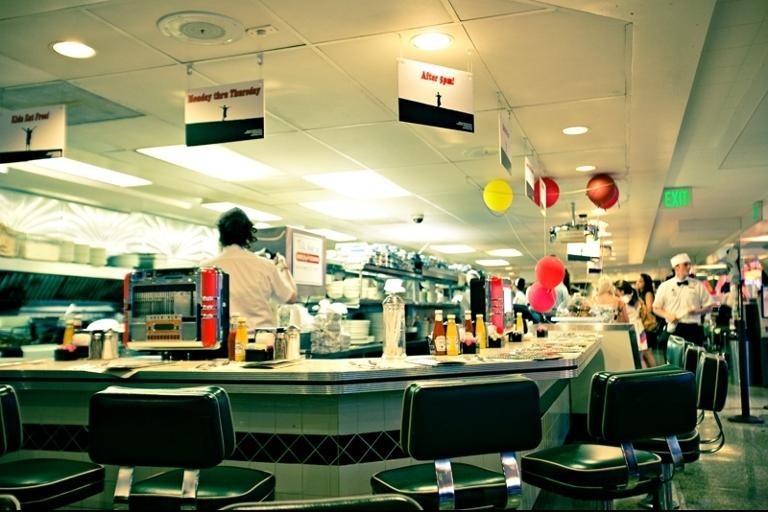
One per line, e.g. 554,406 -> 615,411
600,185 -> 619,209
483,180 -> 512,212
528,256 -> 565,312
587,174 -> 615,207
533,178 -> 559,208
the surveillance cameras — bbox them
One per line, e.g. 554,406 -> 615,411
412,213 -> 424,223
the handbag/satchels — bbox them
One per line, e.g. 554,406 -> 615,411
638,297 -> 657,331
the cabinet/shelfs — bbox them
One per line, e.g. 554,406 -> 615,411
326,265 -> 466,340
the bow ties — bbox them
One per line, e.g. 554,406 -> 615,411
677,280 -> 688,286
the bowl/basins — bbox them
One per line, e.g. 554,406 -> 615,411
325,271 -> 381,302
367,335 -> 376,342
133,253 -> 169,269
91,328 -> 119,362
298,331 -> 313,349
22,344 -> 58,361
60,239 -> 107,267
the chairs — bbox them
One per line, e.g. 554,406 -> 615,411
642,333 -> 730,511
217,490 -> 422,510
0,384 -> 105,512
89,385 -> 277,510
369,372 -> 541,509
521,365 -> 696,512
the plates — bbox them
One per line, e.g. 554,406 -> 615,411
350,339 -> 370,345
108,253 -> 140,268
346,319 -> 372,340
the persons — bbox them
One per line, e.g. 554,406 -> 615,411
512,269 -> 571,317
198,207 -> 298,336
594,253 -> 715,368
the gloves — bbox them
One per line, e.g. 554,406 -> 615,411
274,252 -> 288,271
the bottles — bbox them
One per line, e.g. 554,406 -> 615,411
577,214 -> 588,224
405,313 -> 431,342
226,314 -> 300,361
516,313 -> 524,336
431,308 -> 487,356
369,244 -> 423,274
548,224 -> 599,241
63,320 -> 74,348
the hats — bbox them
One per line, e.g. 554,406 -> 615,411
670,253 -> 691,268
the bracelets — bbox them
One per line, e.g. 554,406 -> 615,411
279,264 -> 288,272
